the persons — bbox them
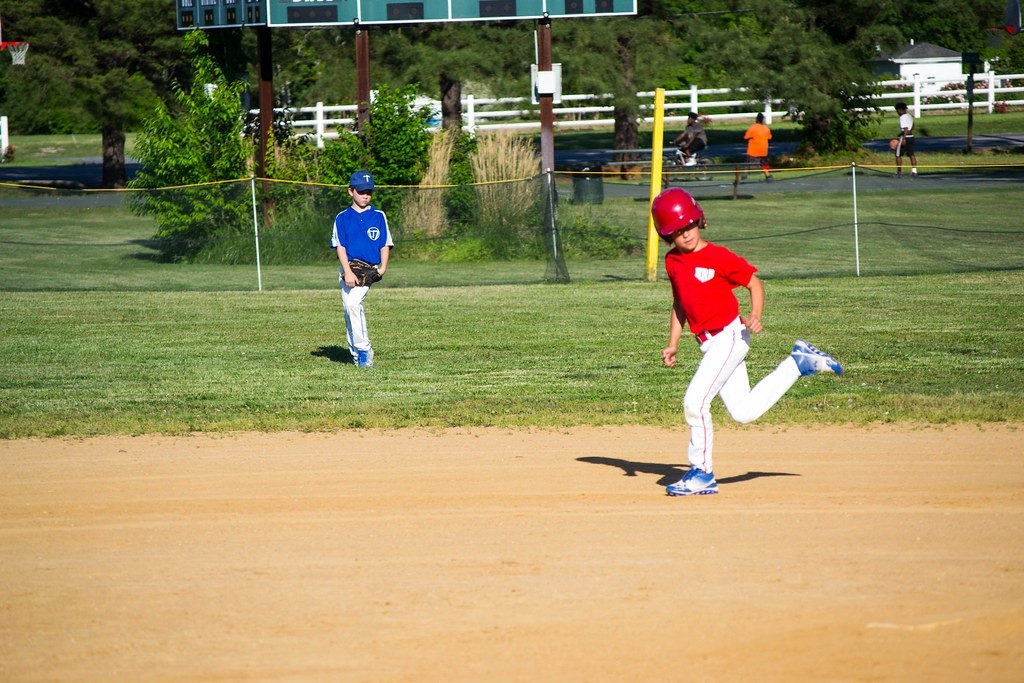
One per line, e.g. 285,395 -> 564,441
651,186 -> 843,497
744,113 -> 774,184
673,112 -> 707,166
330,170 -> 394,370
890,102 -> 917,179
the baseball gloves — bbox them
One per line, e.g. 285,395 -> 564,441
340,257 -> 382,286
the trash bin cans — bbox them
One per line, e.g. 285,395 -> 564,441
572,165 -> 604,204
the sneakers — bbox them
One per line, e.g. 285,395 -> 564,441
357,348 -> 374,371
352,356 -> 358,367
666,466 -> 719,496
790,338 -> 843,376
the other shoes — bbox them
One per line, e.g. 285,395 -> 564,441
685,158 -> 697,166
911,167 -> 917,177
892,173 -> 902,178
767,175 -> 773,183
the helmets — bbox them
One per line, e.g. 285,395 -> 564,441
652,187 -> 707,245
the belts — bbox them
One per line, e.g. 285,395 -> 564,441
695,315 -> 744,344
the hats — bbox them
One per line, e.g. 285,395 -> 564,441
349,171 -> 375,191
895,103 -> 908,109
688,112 -> 697,119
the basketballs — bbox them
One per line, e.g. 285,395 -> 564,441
889,139 -> 899,150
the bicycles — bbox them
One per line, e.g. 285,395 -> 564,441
660,140 -> 716,182
